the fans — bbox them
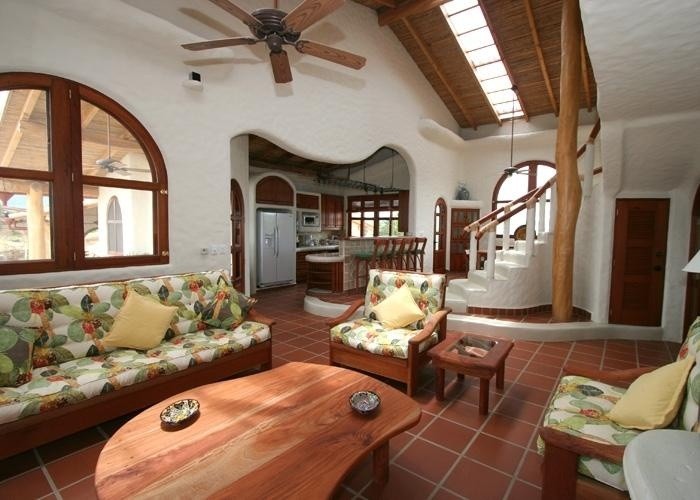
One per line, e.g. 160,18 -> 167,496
180,0 -> 367,83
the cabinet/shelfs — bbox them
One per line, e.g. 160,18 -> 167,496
257,177 -> 294,206
296,193 -> 319,209
321,192 -> 344,231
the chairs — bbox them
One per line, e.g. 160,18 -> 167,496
324,268 -> 453,397
354,236 -> 427,295
534,315 -> 700,499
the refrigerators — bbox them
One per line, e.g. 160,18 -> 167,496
256,209 -> 296,289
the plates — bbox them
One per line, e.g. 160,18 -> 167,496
160,398 -> 200,429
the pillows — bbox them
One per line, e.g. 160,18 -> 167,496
370,281 -> 427,332
0,324 -> 44,389
603,354 -> 695,430
102,289 -> 179,352
194,274 -> 257,332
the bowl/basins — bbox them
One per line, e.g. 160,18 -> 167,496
348,391 -> 382,417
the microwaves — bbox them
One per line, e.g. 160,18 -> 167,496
302,214 -> 320,227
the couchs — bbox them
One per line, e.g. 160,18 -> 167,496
0,269 -> 277,463
